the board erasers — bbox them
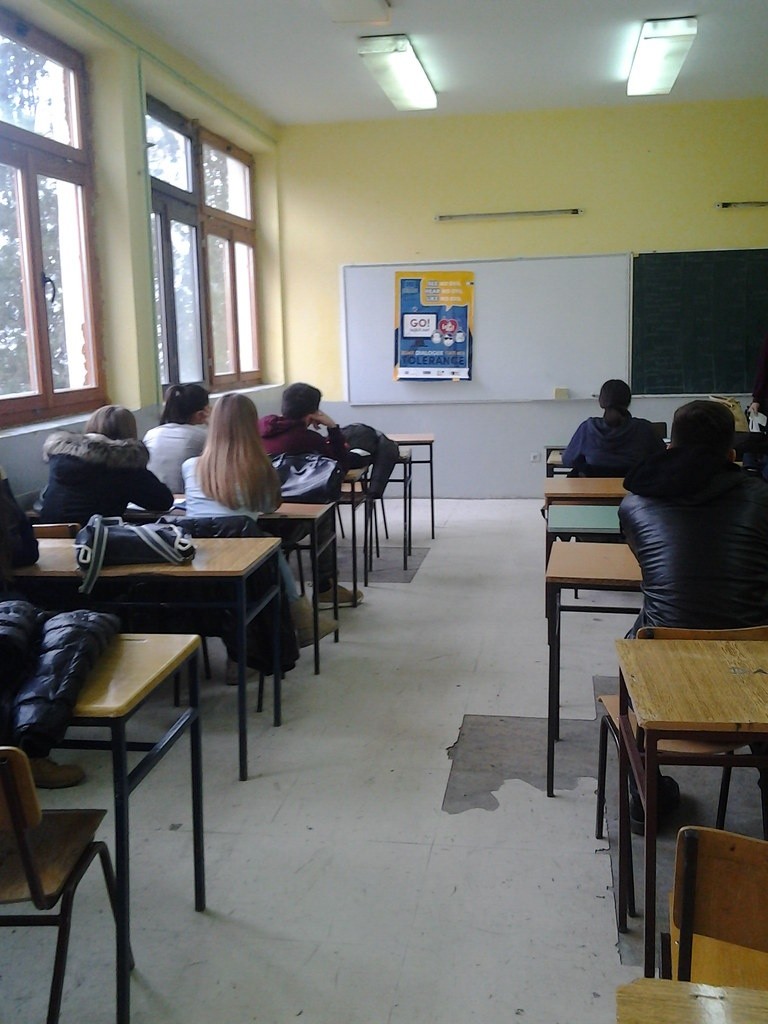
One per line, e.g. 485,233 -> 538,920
555,389 -> 571,401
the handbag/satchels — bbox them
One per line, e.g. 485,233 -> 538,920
270,451 -> 344,504
709,394 -> 749,432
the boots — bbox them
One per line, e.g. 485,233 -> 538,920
224,658 -> 263,687
289,593 -> 348,648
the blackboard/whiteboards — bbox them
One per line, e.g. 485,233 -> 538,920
343,250 -> 633,407
632,248 -> 768,397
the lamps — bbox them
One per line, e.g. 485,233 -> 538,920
322,0 -> 392,24
626,18 -> 699,97
358,36 -> 439,111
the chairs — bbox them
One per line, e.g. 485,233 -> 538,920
592,627 -> 768,917
666,824 -> 768,989
0,514 -> 300,1024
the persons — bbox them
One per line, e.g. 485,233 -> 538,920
0,469 -> 85,788
256,384 -> 364,610
181,394 -> 340,684
561,380 -> 668,545
36,405 -> 173,530
743,335 -> 768,481
142,379 -> 210,494
619,399 -> 768,837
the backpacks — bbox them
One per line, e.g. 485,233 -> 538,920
73,512 -> 196,595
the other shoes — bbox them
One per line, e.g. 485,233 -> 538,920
31,756 -> 86,789
629,775 -> 681,835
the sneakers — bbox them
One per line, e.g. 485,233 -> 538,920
317,577 -> 363,609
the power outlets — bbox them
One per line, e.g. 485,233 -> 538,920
530,453 -> 542,463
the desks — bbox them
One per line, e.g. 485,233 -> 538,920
336,469 -> 369,609
0,599 -> 205,1024
6,538 -> 282,781
546,539 -> 644,798
615,973 -> 768,1024
542,477 -> 628,523
545,446 -> 573,477
617,637 -> 768,979
124,500 -> 339,675
544,504 -> 621,618
372,447 -> 413,571
386,433 -> 435,539
